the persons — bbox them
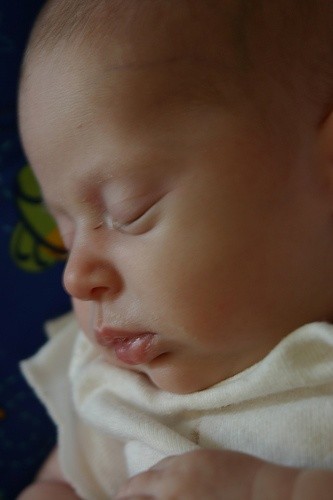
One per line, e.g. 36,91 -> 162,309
19,0 -> 333,500
